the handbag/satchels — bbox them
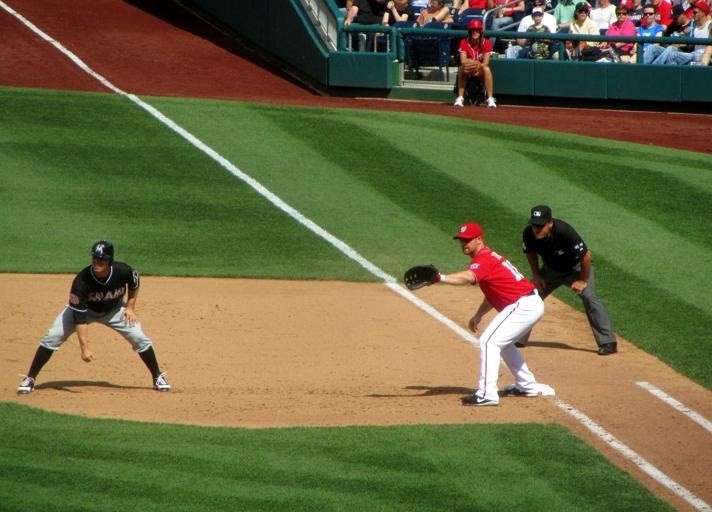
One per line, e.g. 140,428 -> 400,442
582,46 -> 606,61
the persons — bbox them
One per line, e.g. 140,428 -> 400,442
334,0 -> 712,66
402,221 -> 557,408
507,204 -> 619,357
450,19 -> 501,113
12,238 -> 181,401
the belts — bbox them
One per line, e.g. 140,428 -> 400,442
527,291 -> 535,295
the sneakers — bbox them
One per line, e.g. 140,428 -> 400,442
504,384 -> 538,397
454,97 -> 464,107
598,342 -> 617,355
17,374 -> 34,393
487,98 -> 497,108
153,372 -> 171,391
462,395 -> 499,406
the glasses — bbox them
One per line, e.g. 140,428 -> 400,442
534,3 -> 542,6
643,13 -> 654,15
617,12 -> 627,16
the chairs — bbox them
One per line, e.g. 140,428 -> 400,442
345,0 -> 562,80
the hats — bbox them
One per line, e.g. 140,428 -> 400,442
528,205 -> 551,225
576,2 -> 588,10
690,2 -> 709,15
669,4 -> 684,19
91,241 -> 114,259
532,7 -> 543,15
453,223 -> 482,240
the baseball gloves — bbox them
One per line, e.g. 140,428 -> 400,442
463,58 -> 481,74
404,264 -> 440,290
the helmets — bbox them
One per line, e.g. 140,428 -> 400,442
468,19 -> 483,30
